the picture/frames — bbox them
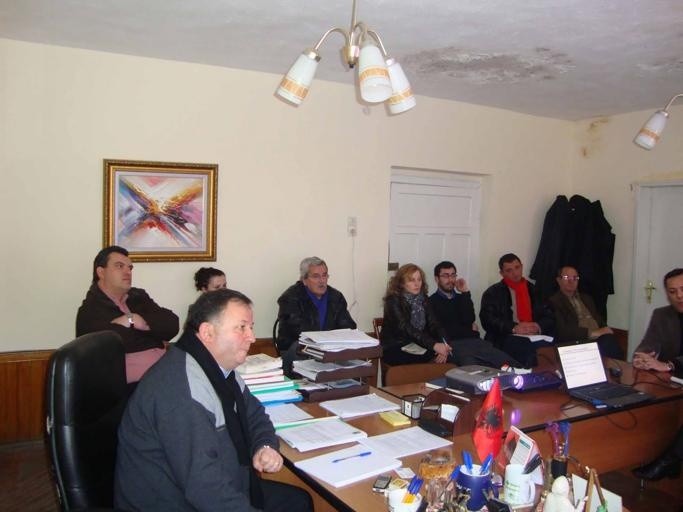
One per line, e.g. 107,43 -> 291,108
100,159 -> 219,261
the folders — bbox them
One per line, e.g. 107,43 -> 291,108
297,344 -> 384,363
293,359 -> 376,382
294,382 -> 370,403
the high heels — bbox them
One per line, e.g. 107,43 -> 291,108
632,454 -> 680,480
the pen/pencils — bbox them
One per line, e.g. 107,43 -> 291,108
410,480 -> 423,504
462,450 -> 471,475
333,452 -> 371,463
402,474 -> 417,503
442,336 -> 453,357
467,452 -> 474,475
524,453 -> 540,471
481,459 -> 493,475
438,465 -> 461,500
546,420 -> 560,457
480,452 -> 493,473
561,422 -> 570,456
525,460 -> 541,475
405,479 -> 420,504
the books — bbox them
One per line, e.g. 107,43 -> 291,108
511,333 -> 554,343
670,375 -> 683,384
233,326 -> 455,488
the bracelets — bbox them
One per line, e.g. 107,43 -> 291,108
513,328 -> 515,333
262,443 -> 272,449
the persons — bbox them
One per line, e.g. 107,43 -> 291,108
276,256 -> 357,354
631,424 -> 682,482
549,266 -> 625,358
478,252 -> 558,369
428,261 -> 524,369
378,262 -> 452,364
74,245 -> 180,394
629,267 -> 683,373
114,289 -> 317,511
184,266 -> 227,336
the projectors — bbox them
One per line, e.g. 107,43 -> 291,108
445,364 -> 524,397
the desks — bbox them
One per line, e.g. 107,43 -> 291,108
251,356 -> 682,512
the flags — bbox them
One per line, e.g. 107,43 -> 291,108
472,377 -> 504,465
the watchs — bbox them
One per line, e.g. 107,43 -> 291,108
665,362 -> 674,371
127,312 -> 135,328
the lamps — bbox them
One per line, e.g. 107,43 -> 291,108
631,93 -> 682,150
274,0 -> 417,119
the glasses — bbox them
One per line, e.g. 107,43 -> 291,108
560,275 -> 581,282
438,273 -> 458,280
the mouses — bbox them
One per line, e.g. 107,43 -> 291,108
608,364 -> 624,379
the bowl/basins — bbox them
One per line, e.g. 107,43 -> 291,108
387,489 -> 421,512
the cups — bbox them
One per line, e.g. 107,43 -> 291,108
504,464 -> 543,504
455,464 -> 498,510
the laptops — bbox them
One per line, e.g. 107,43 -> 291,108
552,339 -> 654,411
